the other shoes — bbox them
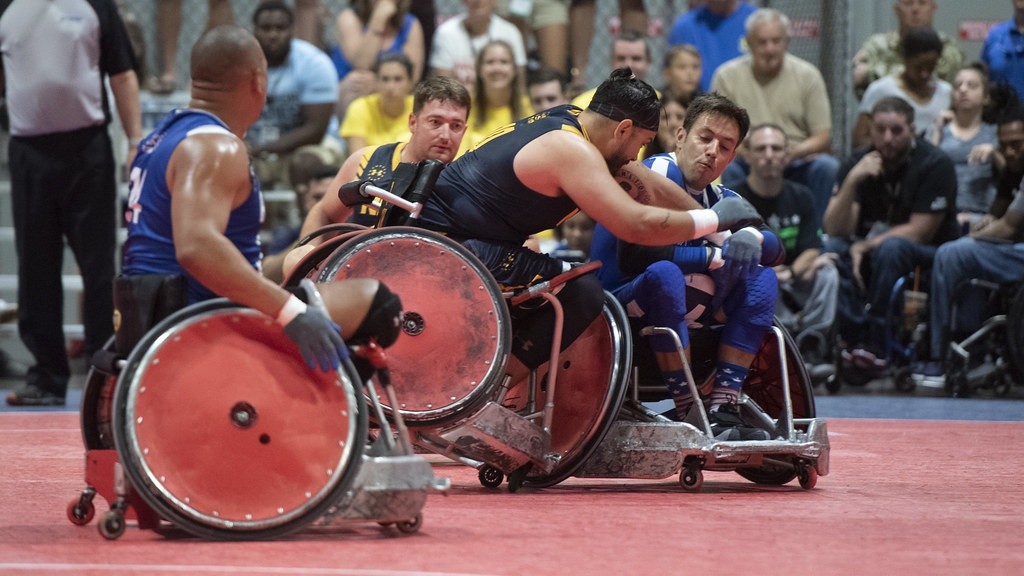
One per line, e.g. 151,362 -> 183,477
852,343 -> 889,366
675,393 -> 771,441
6,385 -> 65,405
801,347 -> 838,373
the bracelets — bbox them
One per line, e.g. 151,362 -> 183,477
126,138 -> 140,150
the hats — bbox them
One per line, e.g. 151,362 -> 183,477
588,65 -> 669,132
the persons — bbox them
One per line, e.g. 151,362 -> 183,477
113,23 -> 406,394
588,91 -> 788,442
241,0 -> 1024,396
0,0 -> 147,408
387,65 -> 765,407
279,73 -> 474,307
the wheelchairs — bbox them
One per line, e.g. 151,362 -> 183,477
290,159 -> 603,488
498,285 -> 830,491
68,272 -> 430,543
806,222 -> 1024,398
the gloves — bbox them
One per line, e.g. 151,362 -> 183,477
712,196 -> 764,235
719,228 -> 762,280
